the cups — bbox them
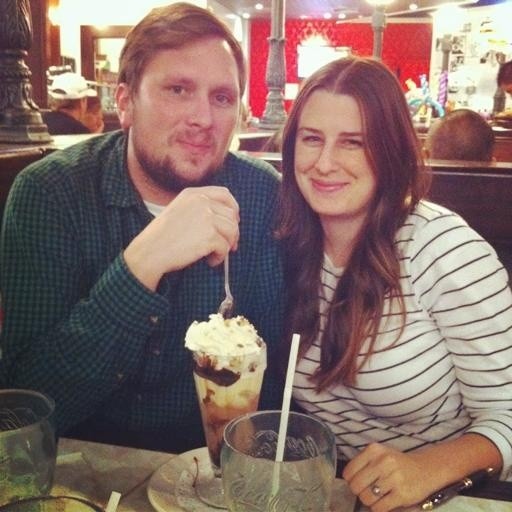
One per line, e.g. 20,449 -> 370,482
193,344 -> 267,509
0,389 -> 58,506
221,409 -> 337,512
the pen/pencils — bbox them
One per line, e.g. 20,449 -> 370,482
421,467 -> 497,511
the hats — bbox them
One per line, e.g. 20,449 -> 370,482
46,72 -> 98,100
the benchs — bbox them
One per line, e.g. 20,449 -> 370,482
49,120 -> 512,285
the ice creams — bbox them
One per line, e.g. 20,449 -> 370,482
183,313 -> 268,466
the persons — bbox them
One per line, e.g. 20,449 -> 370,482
40,72 -> 98,135
0,1 -> 296,455
421,109 -> 497,163
497,60 -> 512,94
273,56 -> 512,511
80,96 -> 103,132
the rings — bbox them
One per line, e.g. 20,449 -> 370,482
371,484 -> 383,497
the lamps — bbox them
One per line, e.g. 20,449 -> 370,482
432,4 -> 469,70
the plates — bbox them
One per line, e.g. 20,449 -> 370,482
147,445 -> 233,512
